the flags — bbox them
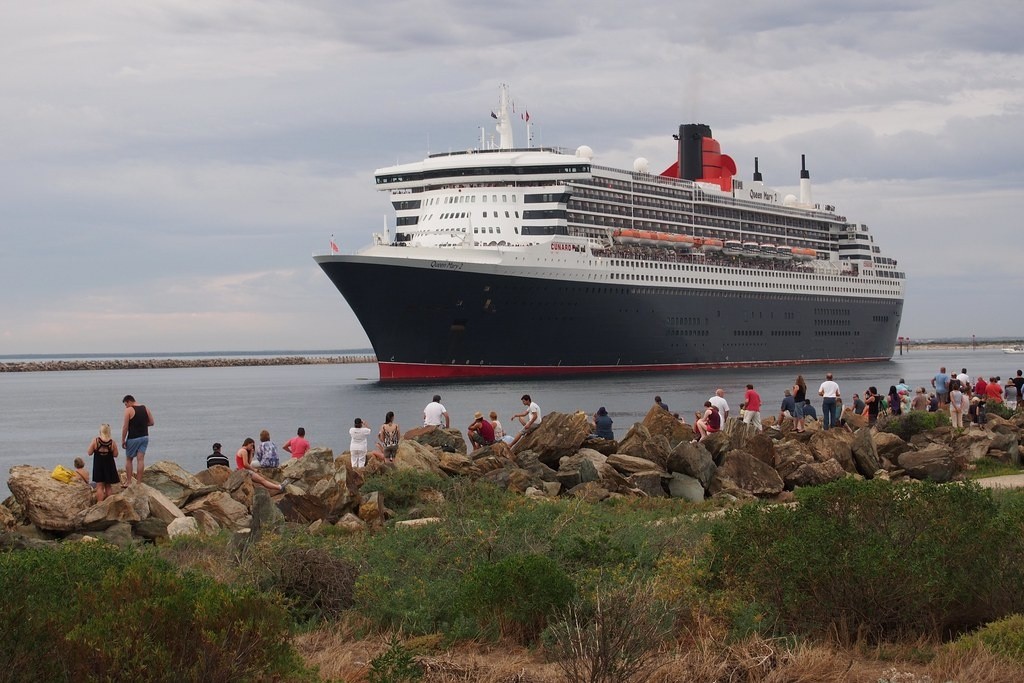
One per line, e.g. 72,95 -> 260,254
330,241 -> 338,251
526,111 -> 530,121
490,112 -> 497,118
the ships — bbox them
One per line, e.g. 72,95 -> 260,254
310,84 -> 905,382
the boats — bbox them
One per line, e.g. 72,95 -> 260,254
613,230 -> 817,261
1002,345 -> 1024,354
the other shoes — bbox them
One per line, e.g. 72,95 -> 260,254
797,430 -> 805,433
790,429 -> 798,432
770,425 -> 780,431
280,480 -> 287,492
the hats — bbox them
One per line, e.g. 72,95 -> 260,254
213,443 -> 222,450
1006,380 -> 1015,385
355,418 -> 363,424
474,411 -> 483,419
597,407 -> 608,416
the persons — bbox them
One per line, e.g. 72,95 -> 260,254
468,411 -> 495,450
349,418 -> 371,467
236,437 -> 288,492
489,412 -> 505,442
372,432 -> 385,462
88,423 -> 118,504
588,407 -> 615,440
674,367 -> 1024,442
207,443 -> 229,468
423,394 -> 449,429
655,396 -> 669,411
507,394 -> 541,448
381,411 -> 400,463
250,430 -> 280,468
122,394 -> 154,488
74,457 -> 89,483
283,427 -> 310,458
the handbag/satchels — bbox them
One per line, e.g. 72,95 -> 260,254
51,465 -> 76,484
